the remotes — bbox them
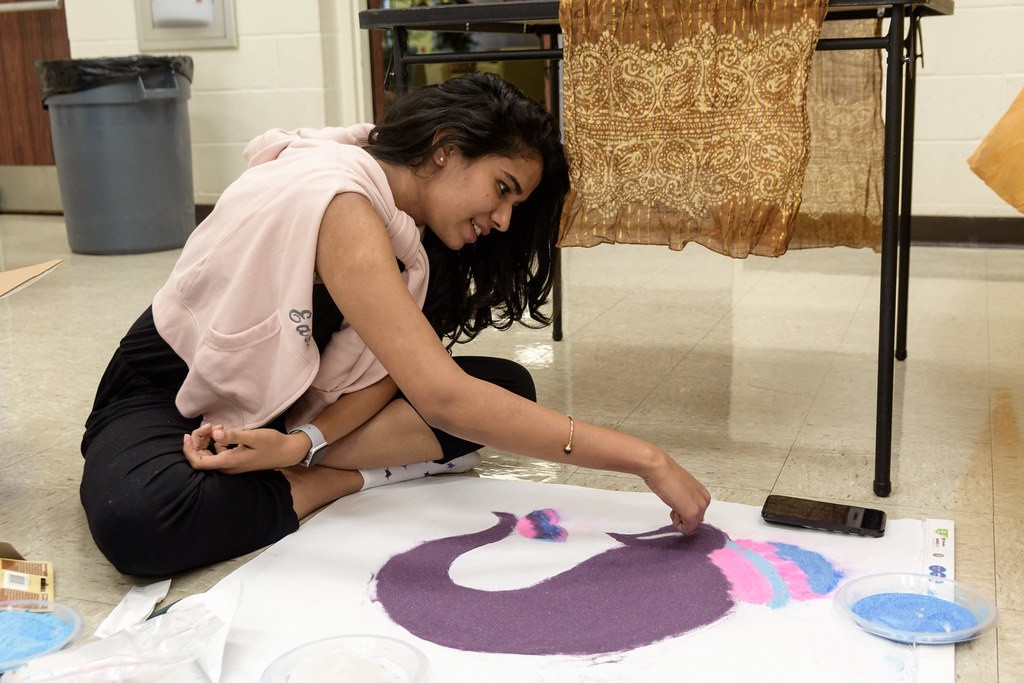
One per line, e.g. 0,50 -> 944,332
761,495 -> 888,538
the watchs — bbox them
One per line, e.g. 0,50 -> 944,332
289,422 -> 328,468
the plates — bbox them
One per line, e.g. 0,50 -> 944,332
0,599 -> 82,674
834,572 -> 1003,645
259,634 -> 429,683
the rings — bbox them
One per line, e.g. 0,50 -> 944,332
699,517 -> 705,523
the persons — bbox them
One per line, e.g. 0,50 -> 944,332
80,72 -> 710,575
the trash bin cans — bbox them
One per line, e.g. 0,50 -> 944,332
34,54 -> 198,257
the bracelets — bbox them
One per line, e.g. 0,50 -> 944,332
563,415 -> 574,454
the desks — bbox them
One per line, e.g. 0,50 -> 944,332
357,0 -> 954,499
